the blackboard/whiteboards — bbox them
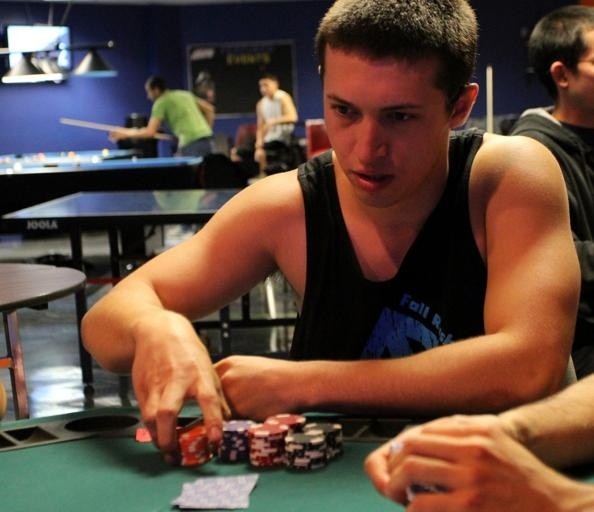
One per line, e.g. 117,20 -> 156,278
185,38 -> 299,120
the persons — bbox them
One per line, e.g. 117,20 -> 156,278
254,73 -> 298,177
503,5 -> 594,380
364,373 -> 594,512
109,74 -> 216,158
78,0 -> 582,463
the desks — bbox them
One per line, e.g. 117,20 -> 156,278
0,259 -> 97,421
0,148 -> 150,262
0,400 -> 431,512
0,184 -> 302,388
0,153 -> 208,273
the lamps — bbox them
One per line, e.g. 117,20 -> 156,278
0,51 -> 52,87
67,44 -> 119,81
31,50 -> 68,87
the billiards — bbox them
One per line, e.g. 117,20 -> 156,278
101,147 -> 109,155
0,151 -> 82,169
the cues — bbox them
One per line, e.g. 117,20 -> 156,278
0,41 -> 113,55
486,66 -> 493,134
59,119 -> 173,142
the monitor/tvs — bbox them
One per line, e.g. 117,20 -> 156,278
4,23 -> 75,77
